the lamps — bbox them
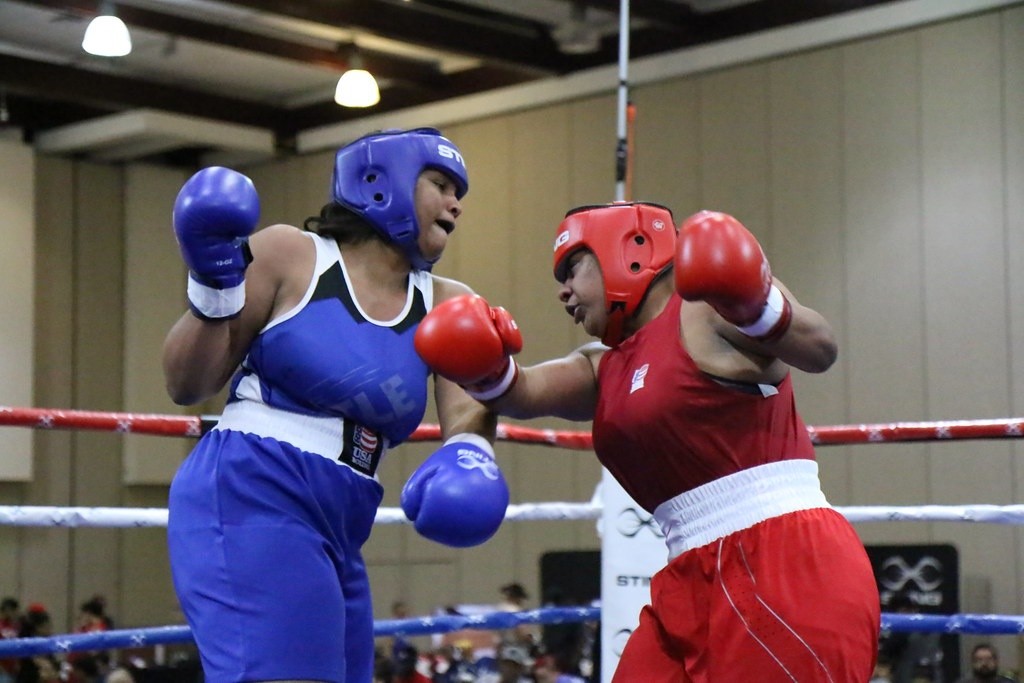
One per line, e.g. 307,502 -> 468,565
82,0 -> 132,58
334,42 -> 380,109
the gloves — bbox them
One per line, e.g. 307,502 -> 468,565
414,295 -> 524,405
173,164 -> 258,323
399,432 -> 511,548
675,210 -> 792,345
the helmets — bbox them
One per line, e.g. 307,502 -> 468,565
553,201 -> 680,348
333,127 -> 470,272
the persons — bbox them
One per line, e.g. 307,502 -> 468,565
0,581 -> 590,683
415,203 -> 883,682
161,127 -> 510,683
958,643 -> 1022,682
